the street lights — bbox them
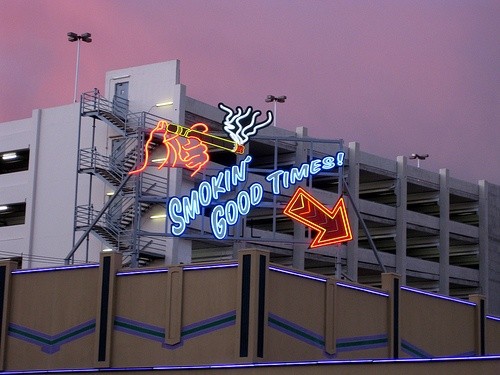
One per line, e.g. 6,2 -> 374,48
66,30 -> 93,102
264,94 -> 288,129
408,151 -> 430,169
